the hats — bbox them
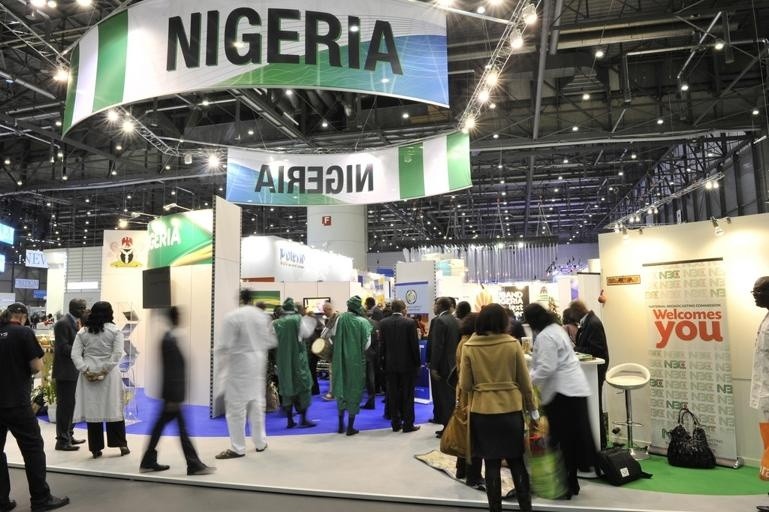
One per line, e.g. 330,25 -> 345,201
348,294 -> 362,313
282,298 -> 296,315
8,302 -> 33,326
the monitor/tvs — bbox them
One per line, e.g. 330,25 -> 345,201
303,298 -> 330,315
142,265 -> 172,310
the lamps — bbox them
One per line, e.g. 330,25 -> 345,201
603,171 -> 733,239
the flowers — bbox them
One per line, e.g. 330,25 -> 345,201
42,379 -> 57,407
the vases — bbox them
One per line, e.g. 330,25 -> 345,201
47,404 -> 57,424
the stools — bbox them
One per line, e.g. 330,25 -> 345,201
604,362 -> 651,461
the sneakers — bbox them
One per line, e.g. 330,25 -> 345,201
70,437 -> 86,445
139,463 -> 170,474
392,424 -> 402,433
403,424 -> 421,434
287,420 -> 297,431
1,497 -> 18,512
298,420 -> 317,430
92,449 -> 102,458
187,466 -> 218,475
30,493 -> 70,512
120,446 -> 130,456
215,449 -> 245,460
347,428 -> 360,436
55,444 -> 79,451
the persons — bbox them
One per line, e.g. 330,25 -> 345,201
752,277 -> 769,512
139,306 -> 217,476
1,302 -> 70,512
70,302 -> 131,458
51,298 -> 87,451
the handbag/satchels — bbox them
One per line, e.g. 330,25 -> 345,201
594,447 -> 653,487
666,408 -> 716,469
440,385 -> 473,458
